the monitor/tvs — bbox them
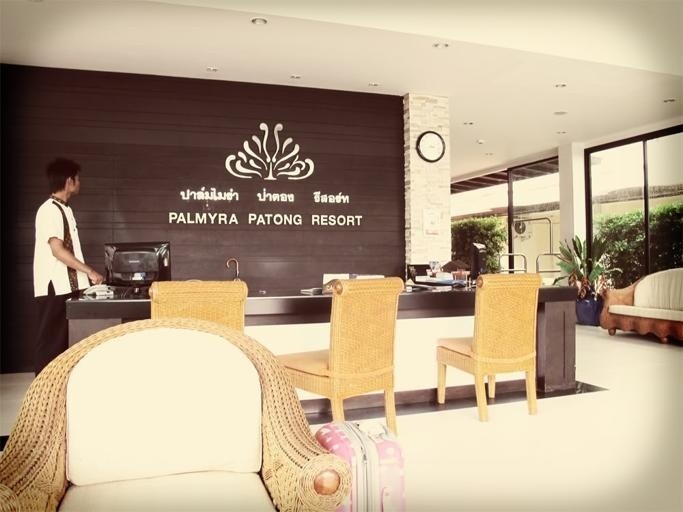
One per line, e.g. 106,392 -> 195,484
470,243 -> 487,279
105,240 -> 170,298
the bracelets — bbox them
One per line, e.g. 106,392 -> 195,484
86,268 -> 95,276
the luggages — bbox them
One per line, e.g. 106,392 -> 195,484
317,421 -> 405,512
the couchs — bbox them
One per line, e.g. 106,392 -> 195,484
600,267 -> 683,343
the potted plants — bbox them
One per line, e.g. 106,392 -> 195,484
553,235 -> 623,325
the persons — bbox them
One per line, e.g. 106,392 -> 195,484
30,156 -> 104,379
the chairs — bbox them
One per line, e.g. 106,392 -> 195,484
0,318 -> 352,512
436,273 -> 542,422
278,277 -> 404,436
149,280 -> 248,332
